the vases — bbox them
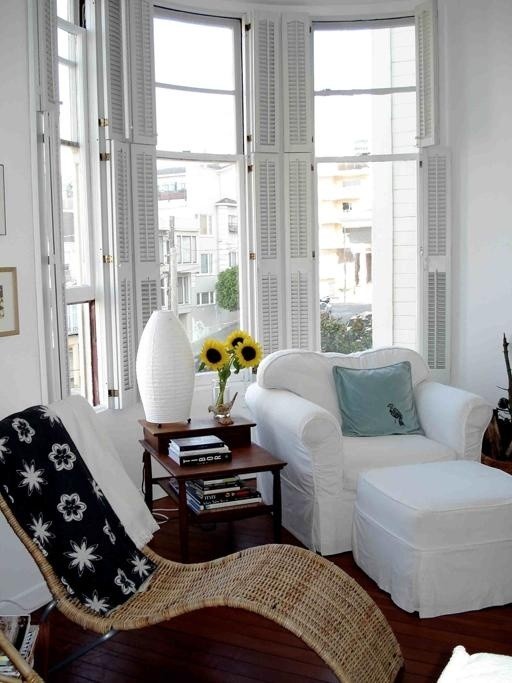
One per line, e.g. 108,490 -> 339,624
211,387 -> 233,420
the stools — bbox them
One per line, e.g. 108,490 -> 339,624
351,462 -> 512,622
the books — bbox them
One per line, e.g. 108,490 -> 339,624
168,447 -> 232,466
168,442 -> 228,455
0,612 -> 40,679
170,434 -> 225,451
168,473 -> 262,514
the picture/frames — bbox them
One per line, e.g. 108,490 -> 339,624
0,265 -> 23,342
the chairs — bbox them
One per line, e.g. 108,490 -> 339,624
243,345 -> 495,555
1,403 -> 404,681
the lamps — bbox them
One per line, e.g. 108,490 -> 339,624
136,309 -> 195,424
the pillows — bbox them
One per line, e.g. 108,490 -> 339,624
329,360 -> 425,441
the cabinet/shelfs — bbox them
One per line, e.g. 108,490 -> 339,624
134,416 -> 289,561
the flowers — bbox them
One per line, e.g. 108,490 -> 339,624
197,332 -> 263,406
